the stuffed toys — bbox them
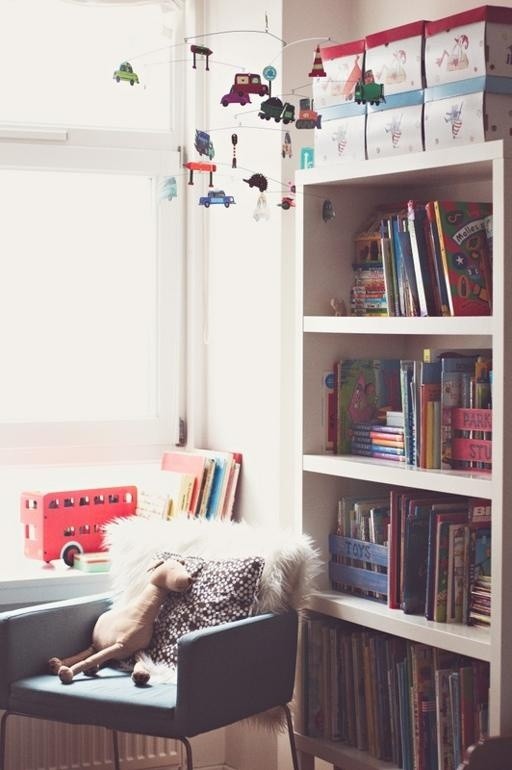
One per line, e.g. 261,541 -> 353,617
45,555 -> 192,686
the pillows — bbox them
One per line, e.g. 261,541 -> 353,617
146,550 -> 264,675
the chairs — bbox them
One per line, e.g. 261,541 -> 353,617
1,515 -> 298,770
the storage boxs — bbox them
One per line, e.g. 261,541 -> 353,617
313,39 -> 366,110
422,3 -> 512,87
364,88 -> 425,159
363,19 -> 430,101
423,74 -> 512,150
312,100 -> 367,167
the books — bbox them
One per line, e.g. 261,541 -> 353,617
301,614 -> 490,769
316,489 -> 491,626
322,348 -> 494,472
137,447 -> 243,522
349,199 -> 494,316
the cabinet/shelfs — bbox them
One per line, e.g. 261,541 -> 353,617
294,141 -> 509,770
